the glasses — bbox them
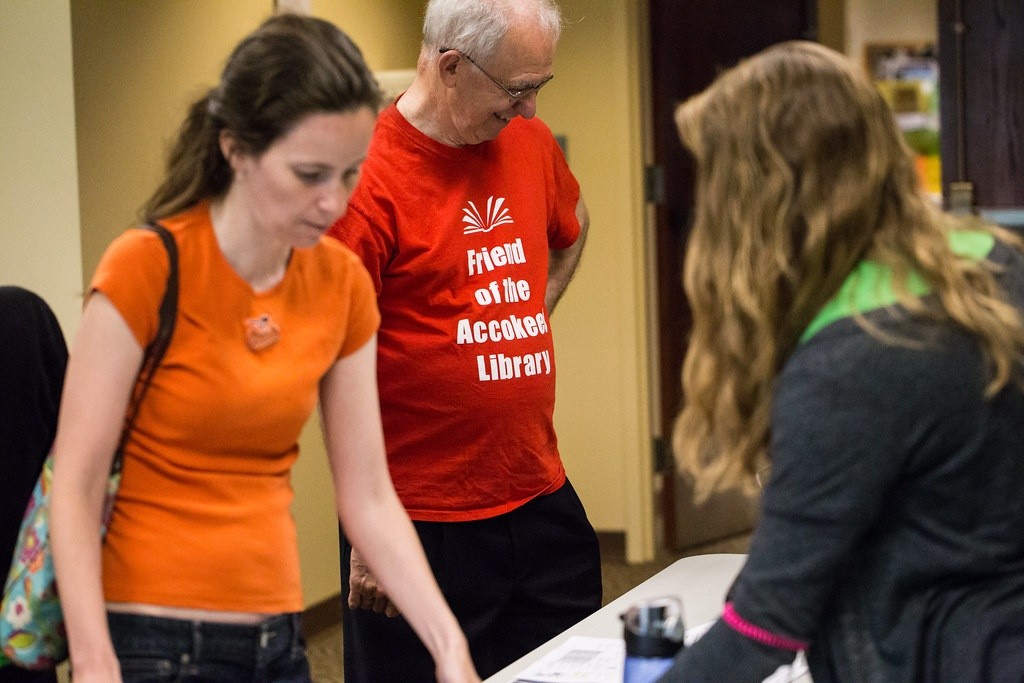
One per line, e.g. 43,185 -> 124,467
435,44 -> 556,100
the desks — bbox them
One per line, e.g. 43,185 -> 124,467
483,552 -> 813,683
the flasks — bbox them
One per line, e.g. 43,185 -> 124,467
619,593 -> 687,683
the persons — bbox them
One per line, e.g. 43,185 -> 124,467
48,13 -> 485,683
652,39 -> 1022,683
325,0 -> 604,683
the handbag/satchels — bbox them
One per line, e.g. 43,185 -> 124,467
0,223 -> 184,672
